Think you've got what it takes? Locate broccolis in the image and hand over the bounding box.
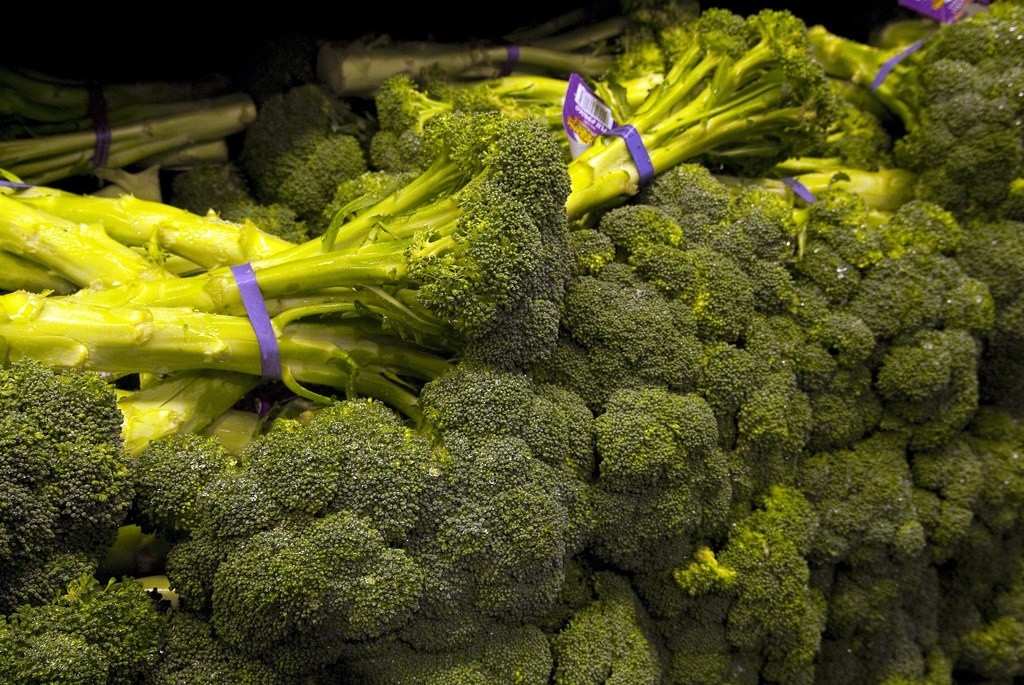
[0,0,1024,685]
